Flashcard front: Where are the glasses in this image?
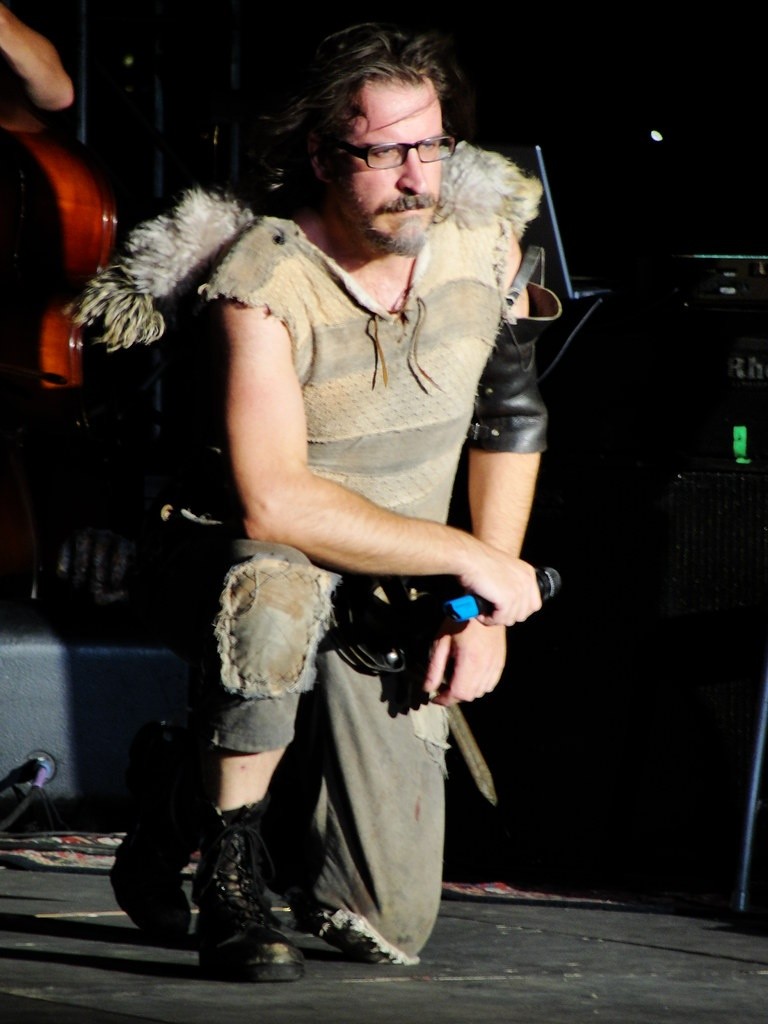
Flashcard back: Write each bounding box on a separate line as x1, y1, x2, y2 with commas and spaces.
331, 129, 456, 170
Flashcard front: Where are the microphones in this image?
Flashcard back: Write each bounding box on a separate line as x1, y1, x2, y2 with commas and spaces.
448, 568, 562, 623
27, 760, 51, 789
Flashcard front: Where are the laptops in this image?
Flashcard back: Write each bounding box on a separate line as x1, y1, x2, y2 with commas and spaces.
505, 145, 610, 300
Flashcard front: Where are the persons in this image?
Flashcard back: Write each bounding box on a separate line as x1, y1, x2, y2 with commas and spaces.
72, 20, 562, 981
0, 1, 75, 134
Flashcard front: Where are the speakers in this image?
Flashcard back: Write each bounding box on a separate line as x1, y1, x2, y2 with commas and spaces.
0, 631, 189, 812
628, 450, 768, 897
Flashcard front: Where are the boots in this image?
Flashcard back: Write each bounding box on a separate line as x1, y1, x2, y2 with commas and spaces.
192, 799, 305, 982
110, 774, 214, 943
